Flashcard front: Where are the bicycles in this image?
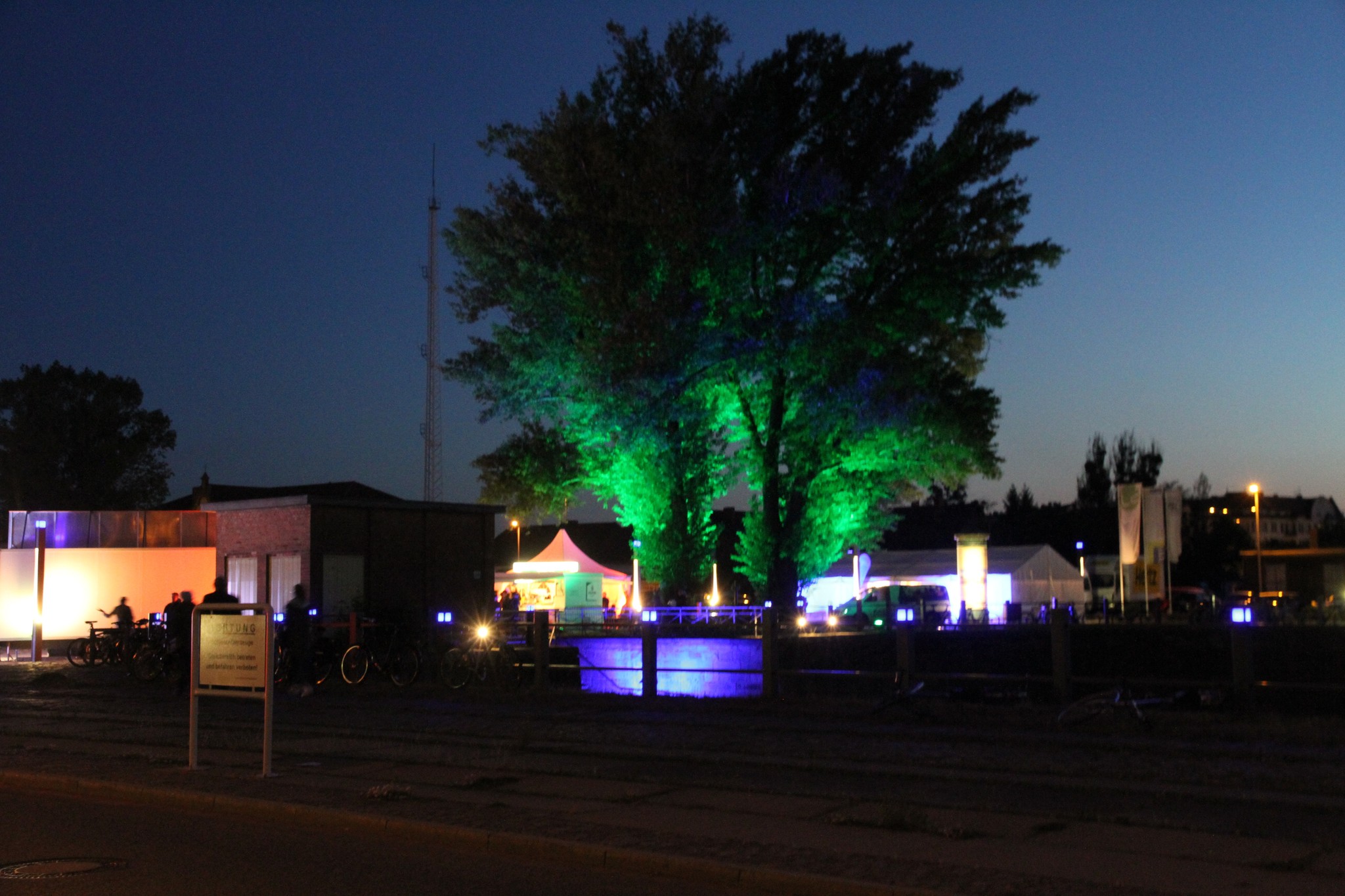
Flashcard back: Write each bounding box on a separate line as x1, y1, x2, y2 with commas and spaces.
66, 619, 525, 691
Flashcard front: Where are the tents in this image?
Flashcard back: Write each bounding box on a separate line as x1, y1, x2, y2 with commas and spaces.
504, 528, 629, 624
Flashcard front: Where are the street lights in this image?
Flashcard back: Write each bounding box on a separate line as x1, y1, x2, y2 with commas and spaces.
1247, 483, 1262, 593
510, 520, 520, 561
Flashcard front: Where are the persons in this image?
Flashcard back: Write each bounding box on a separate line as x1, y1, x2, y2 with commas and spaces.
486, 590, 633, 640
100, 577, 309, 672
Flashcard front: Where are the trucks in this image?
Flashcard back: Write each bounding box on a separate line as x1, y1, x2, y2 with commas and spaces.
1084, 554, 1166, 613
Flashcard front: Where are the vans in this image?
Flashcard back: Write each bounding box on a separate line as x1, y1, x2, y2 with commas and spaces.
832, 585, 952, 629
